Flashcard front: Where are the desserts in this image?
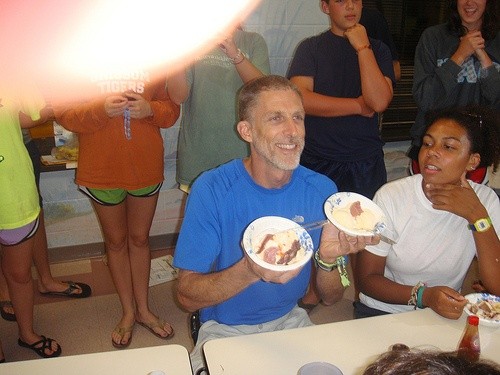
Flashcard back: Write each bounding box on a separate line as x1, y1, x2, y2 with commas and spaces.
332, 201, 379, 232
254, 229, 305, 266
469, 299, 500, 322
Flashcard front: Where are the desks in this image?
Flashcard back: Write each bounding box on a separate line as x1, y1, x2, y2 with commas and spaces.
202, 307, 500, 375
0, 344, 194, 375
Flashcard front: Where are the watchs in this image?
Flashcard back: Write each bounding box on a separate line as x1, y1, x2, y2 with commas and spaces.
467, 216, 492, 232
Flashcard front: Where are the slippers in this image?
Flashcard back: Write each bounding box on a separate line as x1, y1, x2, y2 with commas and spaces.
112, 322, 135, 349
0, 345, 5, 363
40, 281, 92, 297
135, 312, 174, 339
18, 335, 61, 359
0, 300, 17, 322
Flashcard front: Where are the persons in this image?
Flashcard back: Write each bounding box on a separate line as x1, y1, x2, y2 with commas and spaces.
408, 0, 500, 187
0, 90, 84, 362
176, 75, 380, 375
167, 24, 272, 195
289, 0, 394, 200
353, 108, 500, 321
55, 69, 180, 347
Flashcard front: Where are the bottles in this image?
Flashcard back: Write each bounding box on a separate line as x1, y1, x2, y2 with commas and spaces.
456, 315, 481, 362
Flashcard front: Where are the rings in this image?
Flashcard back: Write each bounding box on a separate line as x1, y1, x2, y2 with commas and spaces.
455, 306, 458, 311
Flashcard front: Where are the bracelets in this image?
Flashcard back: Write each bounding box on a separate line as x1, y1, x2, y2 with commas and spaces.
355, 44, 373, 55
230, 48, 245, 64
407, 280, 428, 310
315, 248, 351, 287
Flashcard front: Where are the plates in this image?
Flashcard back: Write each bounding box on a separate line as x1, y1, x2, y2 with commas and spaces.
461, 293, 500, 325
298, 361, 343, 375
244, 215, 313, 271
323, 191, 387, 238
